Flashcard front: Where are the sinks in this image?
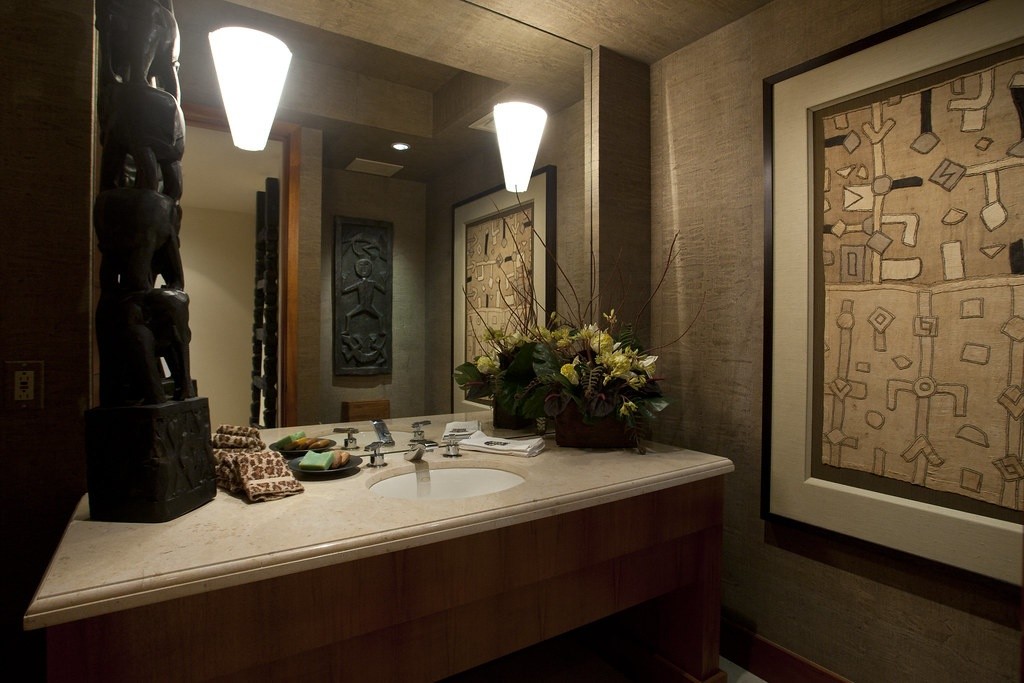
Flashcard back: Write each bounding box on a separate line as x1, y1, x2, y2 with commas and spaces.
317, 429, 418, 458
363, 453, 533, 501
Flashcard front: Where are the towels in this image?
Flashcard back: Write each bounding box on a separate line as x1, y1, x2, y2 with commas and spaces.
218, 452, 306, 502
441, 420, 482, 443
459, 430, 546, 458
212, 423, 266, 486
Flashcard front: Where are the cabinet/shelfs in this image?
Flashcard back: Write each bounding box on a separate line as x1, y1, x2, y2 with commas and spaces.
43, 474, 728, 683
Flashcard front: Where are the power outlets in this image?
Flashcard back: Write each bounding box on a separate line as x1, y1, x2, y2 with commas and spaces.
3, 360, 46, 417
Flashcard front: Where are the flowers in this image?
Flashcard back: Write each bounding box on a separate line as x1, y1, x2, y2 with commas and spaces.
532, 308, 669, 455
452, 320, 565, 417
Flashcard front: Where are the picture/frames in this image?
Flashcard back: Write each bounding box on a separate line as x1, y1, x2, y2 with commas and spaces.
450, 165, 558, 413
760, 0, 1023, 586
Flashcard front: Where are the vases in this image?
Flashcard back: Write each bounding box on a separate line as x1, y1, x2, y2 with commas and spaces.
555, 405, 638, 447
493, 395, 537, 429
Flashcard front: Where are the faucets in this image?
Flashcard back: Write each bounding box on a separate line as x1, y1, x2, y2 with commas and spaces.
404, 421, 439, 461
369, 418, 396, 446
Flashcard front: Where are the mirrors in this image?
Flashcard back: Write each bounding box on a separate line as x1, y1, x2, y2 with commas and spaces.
99, 0, 591, 433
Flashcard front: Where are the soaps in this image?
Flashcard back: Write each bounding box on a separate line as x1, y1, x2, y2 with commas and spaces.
275, 429, 330, 449
298, 450, 350, 470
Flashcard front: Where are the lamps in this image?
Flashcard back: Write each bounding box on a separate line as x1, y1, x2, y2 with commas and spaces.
490, 102, 548, 194
209, 22, 294, 153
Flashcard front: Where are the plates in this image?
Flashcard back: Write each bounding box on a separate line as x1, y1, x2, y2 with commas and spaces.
287, 454, 363, 475
